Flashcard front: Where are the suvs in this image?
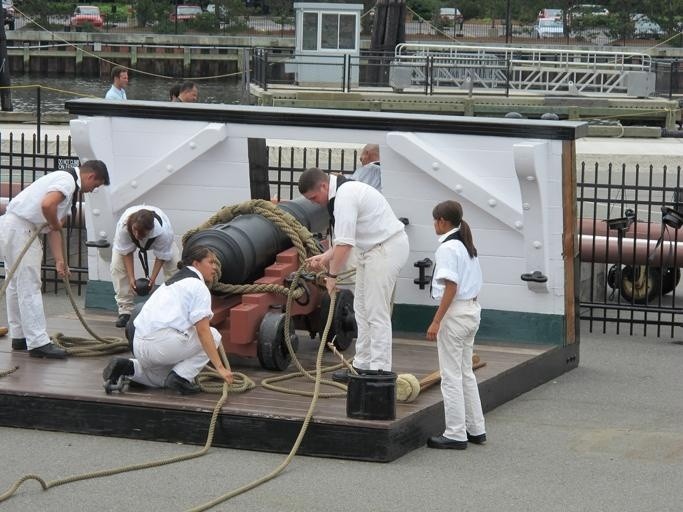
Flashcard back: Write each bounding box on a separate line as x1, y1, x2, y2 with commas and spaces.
65, 5, 103, 32
2, 0, 15, 31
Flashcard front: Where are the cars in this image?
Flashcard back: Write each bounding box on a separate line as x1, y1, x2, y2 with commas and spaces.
168, 3, 230, 30
531, 5, 683, 46
430, 7, 462, 30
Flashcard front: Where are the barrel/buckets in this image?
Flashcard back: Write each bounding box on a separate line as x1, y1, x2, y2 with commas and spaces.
346, 366, 399, 420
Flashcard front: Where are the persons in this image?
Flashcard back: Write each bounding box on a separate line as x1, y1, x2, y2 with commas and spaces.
0, 160, 110, 358
427, 201, 486, 448
103, 248, 233, 394
109, 204, 178, 326
175, 81, 198, 102
170, 83, 182, 101
299, 168, 410, 380
351, 143, 381, 193
105, 65, 128, 100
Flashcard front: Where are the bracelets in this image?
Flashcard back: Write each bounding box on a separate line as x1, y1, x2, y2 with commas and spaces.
326, 272, 338, 279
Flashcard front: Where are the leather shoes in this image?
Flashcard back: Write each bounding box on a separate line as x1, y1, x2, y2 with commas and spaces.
29, 343, 65, 359
332, 367, 361, 383
12, 338, 27, 350
116, 314, 130, 327
103, 358, 123, 381
467, 432, 486, 444
427, 436, 467, 450
164, 374, 201, 394
